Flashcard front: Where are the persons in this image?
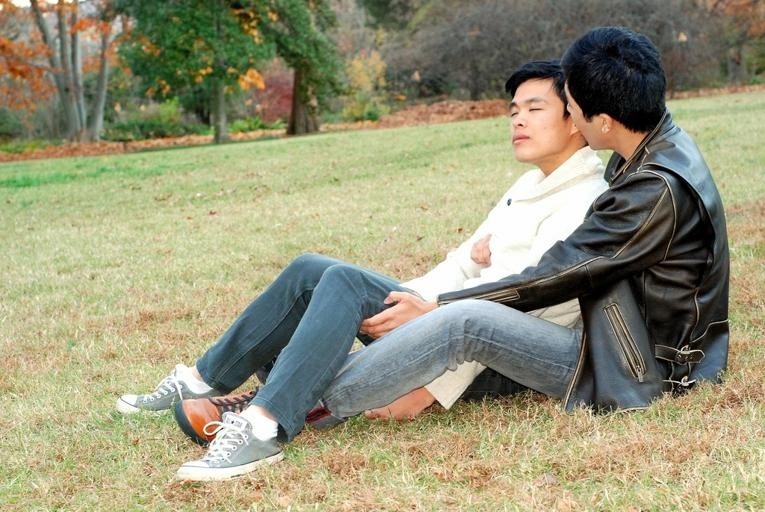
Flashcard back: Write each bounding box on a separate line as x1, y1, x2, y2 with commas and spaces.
114, 57, 614, 482
172, 23, 733, 448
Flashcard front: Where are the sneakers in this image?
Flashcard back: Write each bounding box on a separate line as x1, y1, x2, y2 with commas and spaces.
175, 387, 263, 449
117, 364, 223, 416
177, 412, 285, 481
